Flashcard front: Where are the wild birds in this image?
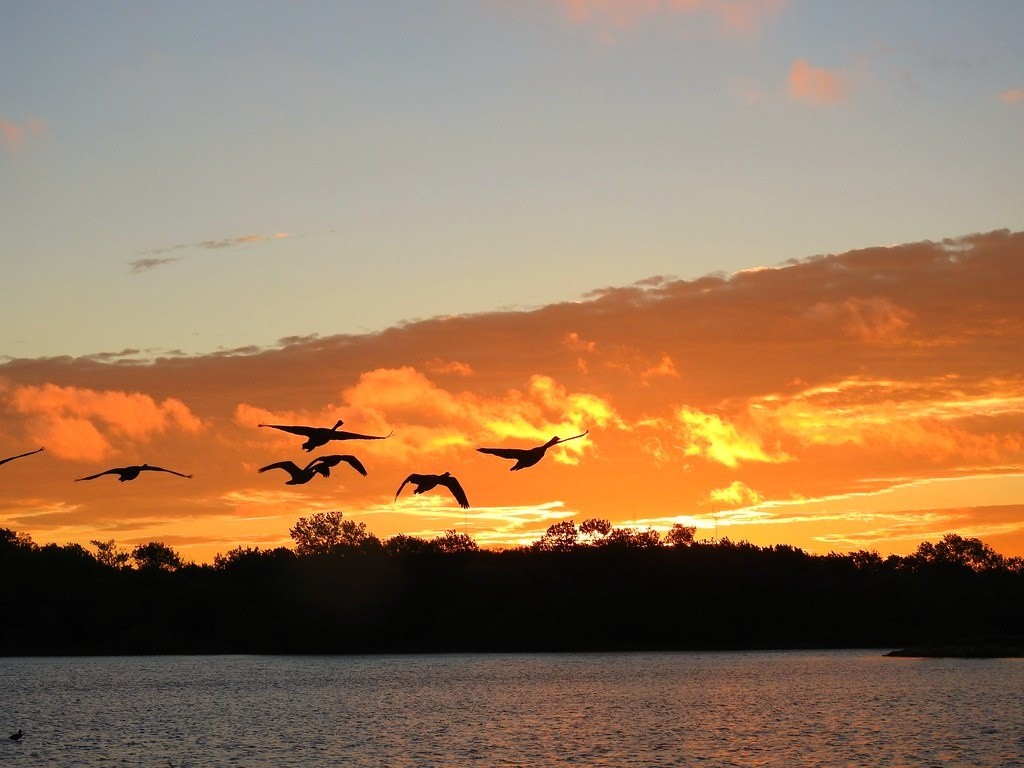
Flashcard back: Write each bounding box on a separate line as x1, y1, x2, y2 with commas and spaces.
0, 446, 45, 464
8, 729, 24, 740
74, 464, 192, 482
394, 471, 470, 510
258, 419, 396, 452
303, 455, 368, 477
474, 429, 591, 472
258, 460, 331, 487
